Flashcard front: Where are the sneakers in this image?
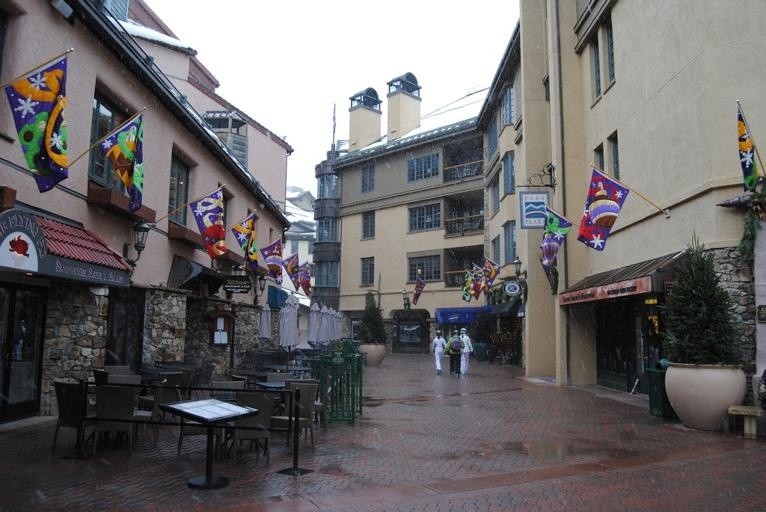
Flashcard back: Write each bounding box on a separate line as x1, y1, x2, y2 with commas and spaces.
450, 372, 454, 375
436, 370, 439, 375
456, 374, 460, 378
439, 370, 442, 373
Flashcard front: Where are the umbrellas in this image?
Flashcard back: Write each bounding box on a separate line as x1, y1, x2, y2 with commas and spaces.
257, 290, 344, 369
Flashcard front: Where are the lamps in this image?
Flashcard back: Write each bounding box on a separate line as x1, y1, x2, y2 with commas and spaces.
254, 270, 270, 296
123, 219, 150, 265
513, 256, 522, 275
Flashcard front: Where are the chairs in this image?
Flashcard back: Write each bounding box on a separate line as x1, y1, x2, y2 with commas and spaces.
52, 364, 319, 489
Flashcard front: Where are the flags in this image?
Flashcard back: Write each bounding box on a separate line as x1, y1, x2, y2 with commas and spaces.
5, 53, 68, 192
737, 105, 762, 194
231, 213, 262, 270
259, 238, 283, 285
188, 187, 228, 259
466, 264, 483, 300
574, 167, 629, 251
96, 110, 143, 213
460, 269, 473, 302
480, 258, 502, 293
538, 207, 572, 297
411, 274, 425, 306
299, 262, 311, 296
281, 252, 300, 291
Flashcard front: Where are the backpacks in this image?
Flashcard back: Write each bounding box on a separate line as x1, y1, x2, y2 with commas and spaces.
450, 337, 461, 352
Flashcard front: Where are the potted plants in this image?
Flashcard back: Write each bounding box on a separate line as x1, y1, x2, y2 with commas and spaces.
658, 229, 748, 434
357, 289, 387, 367
642, 316, 668, 416
469, 308, 493, 361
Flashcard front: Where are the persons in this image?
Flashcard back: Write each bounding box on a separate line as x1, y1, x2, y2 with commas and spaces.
444, 329, 466, 380
431, 330, 448, 376
459, 327, 474, 376
